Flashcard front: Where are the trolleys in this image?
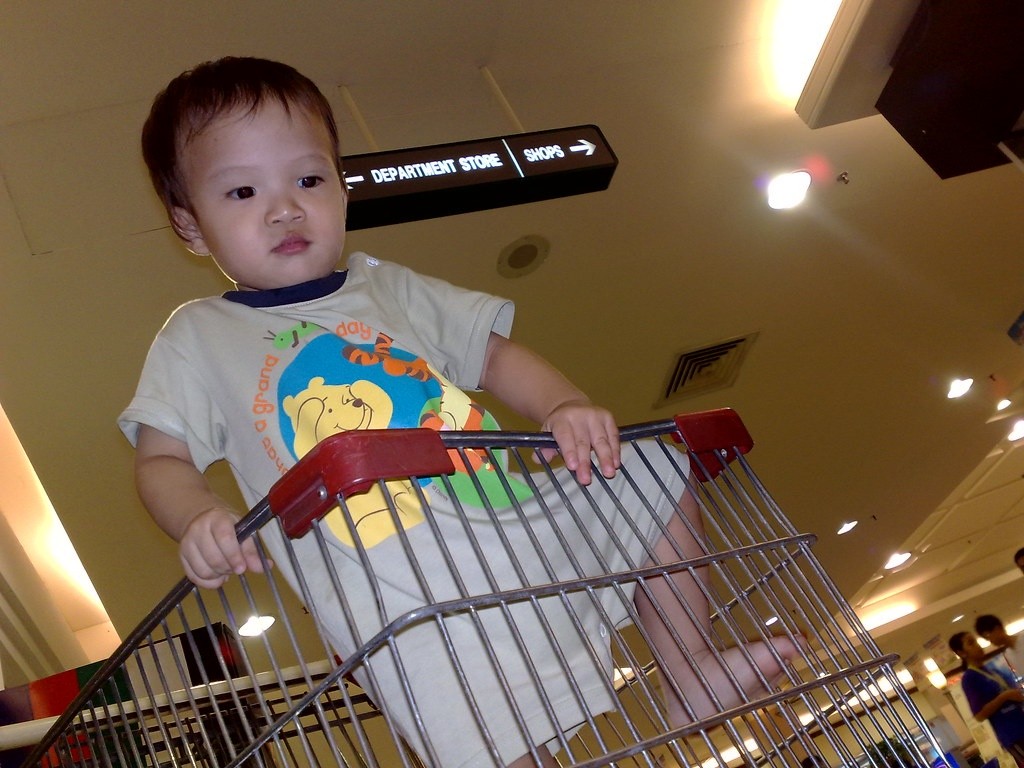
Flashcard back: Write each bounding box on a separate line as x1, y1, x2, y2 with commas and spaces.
18, 406, 953, 768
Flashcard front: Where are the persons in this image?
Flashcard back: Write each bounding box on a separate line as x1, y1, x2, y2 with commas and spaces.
802, 755, 827, 768
115, 56, 805, 768
1013, 546, 1024, 571
972, 614, 1024, 677
947, 631, 1024, 768
